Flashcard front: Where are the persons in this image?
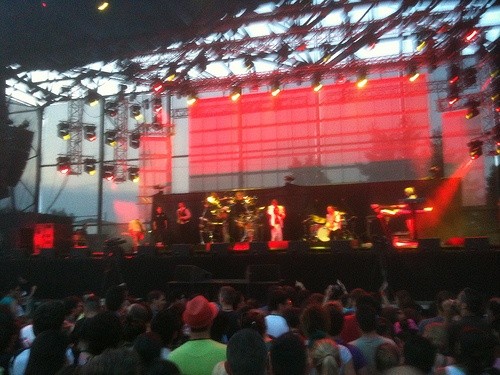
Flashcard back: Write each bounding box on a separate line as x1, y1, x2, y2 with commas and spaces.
0, 284, 500, 375
404, 187, 419, 198
324, 204, 337, 223
126, 198, 235, 247
265, 199, 286, 242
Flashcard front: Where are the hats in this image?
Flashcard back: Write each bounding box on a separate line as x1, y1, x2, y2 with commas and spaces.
182, 296, 219, 327
264, 315, 289, 338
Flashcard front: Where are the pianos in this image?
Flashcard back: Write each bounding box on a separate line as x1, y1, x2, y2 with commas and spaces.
373, 204, 434, 239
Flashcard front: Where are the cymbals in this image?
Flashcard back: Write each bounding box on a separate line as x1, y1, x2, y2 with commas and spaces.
332, 211, 347, 215
308, 214, 327, 222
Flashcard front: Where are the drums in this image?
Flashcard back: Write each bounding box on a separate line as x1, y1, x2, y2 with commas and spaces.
317, 228, 331, 241
308, 224, 322, 237
327, 217, 341, 231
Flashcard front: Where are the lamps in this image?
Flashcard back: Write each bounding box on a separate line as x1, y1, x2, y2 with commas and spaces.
58, 0, 500, 185
